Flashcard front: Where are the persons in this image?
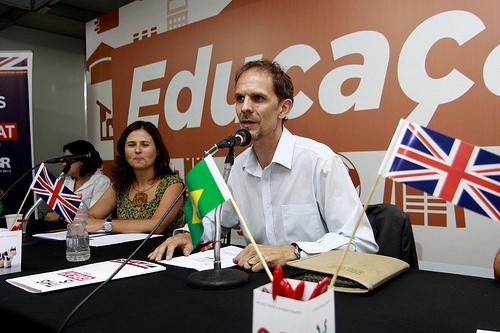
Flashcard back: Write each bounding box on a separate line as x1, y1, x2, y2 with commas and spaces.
86, 121, 186, 235
148, 60, 379, 272
45, 140, 112, 221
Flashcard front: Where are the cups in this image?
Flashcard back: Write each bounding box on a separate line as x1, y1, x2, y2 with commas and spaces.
5, 214, 23, 233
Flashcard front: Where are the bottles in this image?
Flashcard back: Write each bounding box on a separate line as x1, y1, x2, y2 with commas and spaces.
65, 193, 91, 262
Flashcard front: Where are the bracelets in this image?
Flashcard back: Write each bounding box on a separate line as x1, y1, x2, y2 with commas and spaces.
58, 216, 63, 220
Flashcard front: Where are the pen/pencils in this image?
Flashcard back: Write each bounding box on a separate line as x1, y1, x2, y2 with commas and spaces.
310, 276, 330, 301
270, 263, 306, 301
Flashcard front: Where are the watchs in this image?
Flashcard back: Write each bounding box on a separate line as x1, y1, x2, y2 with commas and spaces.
103, 219, 113, 233
291, 243, 302, 259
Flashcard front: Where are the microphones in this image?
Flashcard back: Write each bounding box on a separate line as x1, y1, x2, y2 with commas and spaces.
202, 128, 252, 160
44, 151, 95, 163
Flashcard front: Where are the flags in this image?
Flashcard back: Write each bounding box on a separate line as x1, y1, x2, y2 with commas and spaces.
32, 166, 82, 223
379, 119, 500, 222
184, 156, 232, 247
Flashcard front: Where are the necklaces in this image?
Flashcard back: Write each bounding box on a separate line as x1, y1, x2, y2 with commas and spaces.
132, 179, 157, 207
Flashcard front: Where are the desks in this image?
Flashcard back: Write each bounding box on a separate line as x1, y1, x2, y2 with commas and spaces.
0, 225, 500, 333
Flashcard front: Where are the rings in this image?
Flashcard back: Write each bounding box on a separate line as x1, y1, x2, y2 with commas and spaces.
248, 262, 255, 266
